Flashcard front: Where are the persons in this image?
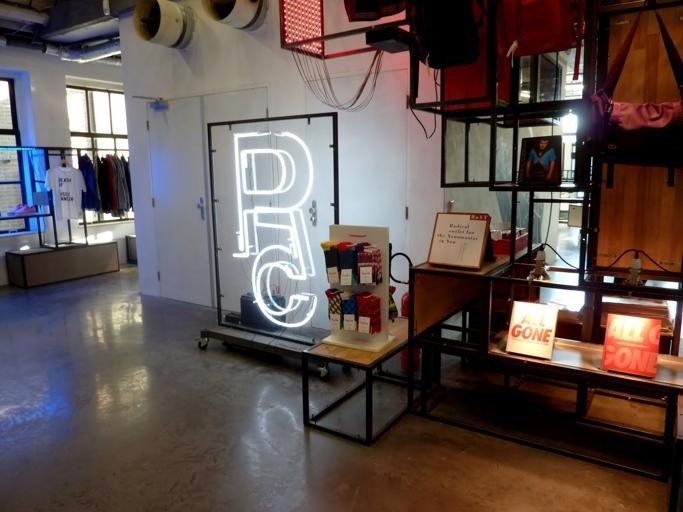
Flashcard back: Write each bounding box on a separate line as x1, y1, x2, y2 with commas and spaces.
523, 136, 557, 183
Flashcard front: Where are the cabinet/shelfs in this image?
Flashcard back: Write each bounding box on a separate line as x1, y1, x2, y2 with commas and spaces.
300, 317, 420, 447
405, 1, 682, 484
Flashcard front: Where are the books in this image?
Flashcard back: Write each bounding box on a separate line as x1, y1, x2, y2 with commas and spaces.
504, 299, 560, 361
598, 313, 662, 379
599, 296, 671, 333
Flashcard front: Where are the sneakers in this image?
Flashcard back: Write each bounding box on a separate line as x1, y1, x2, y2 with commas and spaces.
7, 204, 23, 214
15, 205, 36, 214
